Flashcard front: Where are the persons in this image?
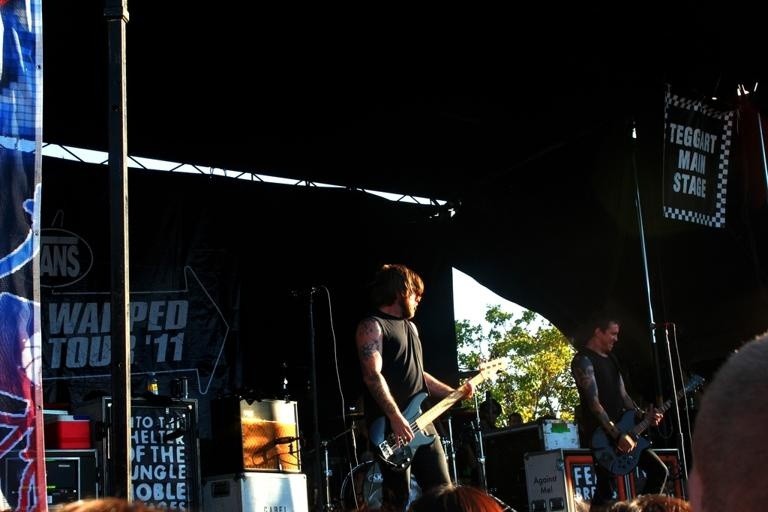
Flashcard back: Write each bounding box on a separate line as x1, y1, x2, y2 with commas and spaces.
413, 485, 507, 512
57, 498, 166, 512
688, 331, 768, 512
509, 413, 523, 426
609, 493, 690, 512
462, 399, 502, 484
356, 264, 477, 512
571, 313, 668, 512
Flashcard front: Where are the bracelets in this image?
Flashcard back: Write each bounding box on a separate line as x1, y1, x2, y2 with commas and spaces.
616, 432, 622, 444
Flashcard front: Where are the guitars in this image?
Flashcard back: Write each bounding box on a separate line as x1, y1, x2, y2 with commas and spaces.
590, 374, 706, 475
369, 358, 507, 473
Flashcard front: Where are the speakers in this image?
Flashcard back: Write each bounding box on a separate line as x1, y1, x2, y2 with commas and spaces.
106, 396, 199, 512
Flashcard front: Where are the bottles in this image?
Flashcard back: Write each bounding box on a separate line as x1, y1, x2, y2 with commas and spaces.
147, 372, 159, 395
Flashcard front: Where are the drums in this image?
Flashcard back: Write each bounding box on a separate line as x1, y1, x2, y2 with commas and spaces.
340, 460, 423, 512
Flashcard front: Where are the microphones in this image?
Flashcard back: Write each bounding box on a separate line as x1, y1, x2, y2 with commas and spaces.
649, 323, 674, 330
286, 285, 322, 299
